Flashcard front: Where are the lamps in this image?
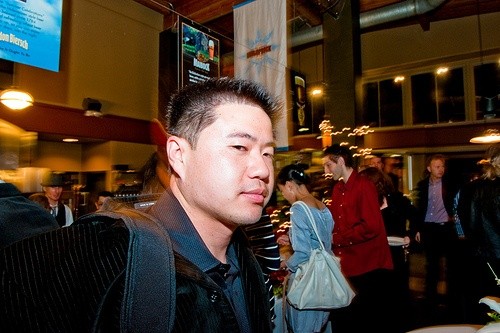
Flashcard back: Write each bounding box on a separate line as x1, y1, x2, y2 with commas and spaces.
469, 125, 500, 144
82, 99, 102, 118
1, 61, 34, 112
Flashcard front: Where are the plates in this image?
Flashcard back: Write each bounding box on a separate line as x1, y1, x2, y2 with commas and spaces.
387, 237, 408, 246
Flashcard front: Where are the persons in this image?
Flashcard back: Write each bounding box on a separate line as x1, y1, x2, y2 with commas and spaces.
369, 157, 385, 170
242, 208, 280, 325
453, 172, 480, 238
42, 173, 74, 227
359, 167, 420, 332
322, 145, 393, 332
357, 165, 369, 174
91, 191, 114, 212
415, 154, 462, 316
0, 79, 283, 333
0, 179, 60, 243
277, 162, 335, 333
388, 163, 404, 189
458, 142, 500, 325
29, 194, 51, 215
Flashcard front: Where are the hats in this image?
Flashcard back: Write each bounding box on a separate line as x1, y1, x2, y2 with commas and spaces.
40, 172, 65, 186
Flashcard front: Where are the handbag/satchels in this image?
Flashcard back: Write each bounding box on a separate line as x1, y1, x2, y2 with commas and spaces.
286, 249, 356, 309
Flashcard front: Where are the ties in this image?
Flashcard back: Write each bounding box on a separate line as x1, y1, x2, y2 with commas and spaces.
52, 207, 56, 217
